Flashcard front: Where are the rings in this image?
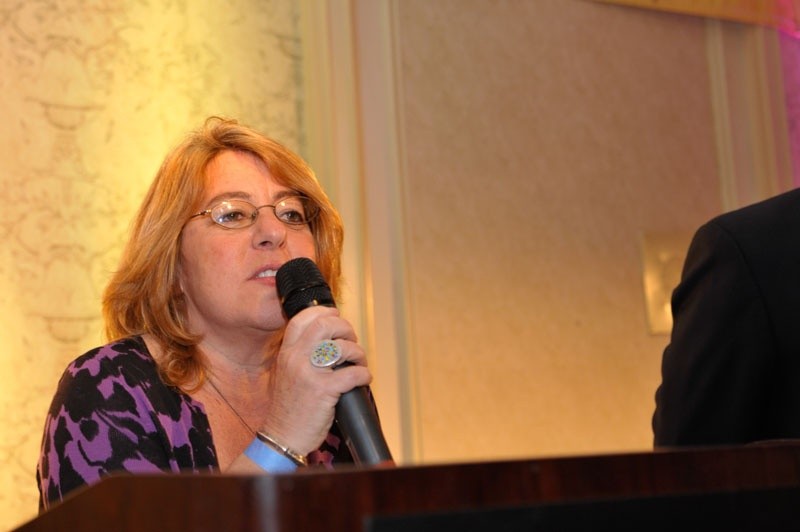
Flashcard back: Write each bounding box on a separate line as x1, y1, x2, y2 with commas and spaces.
311, 339, 341, 368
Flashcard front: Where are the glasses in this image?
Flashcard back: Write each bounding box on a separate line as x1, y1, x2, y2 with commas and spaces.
189, 196, 320, 229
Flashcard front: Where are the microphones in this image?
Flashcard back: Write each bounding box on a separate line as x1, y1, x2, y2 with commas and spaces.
276, 257, 395, 469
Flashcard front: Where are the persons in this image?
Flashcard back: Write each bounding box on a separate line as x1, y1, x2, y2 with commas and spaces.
36, 115, 357, 515
652, 188, 800, 453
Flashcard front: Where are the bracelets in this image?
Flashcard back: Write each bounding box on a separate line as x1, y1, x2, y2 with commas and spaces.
255, 430, 307, 467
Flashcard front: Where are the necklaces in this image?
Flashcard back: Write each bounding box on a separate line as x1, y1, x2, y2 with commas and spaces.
205, 374, 256, 436
